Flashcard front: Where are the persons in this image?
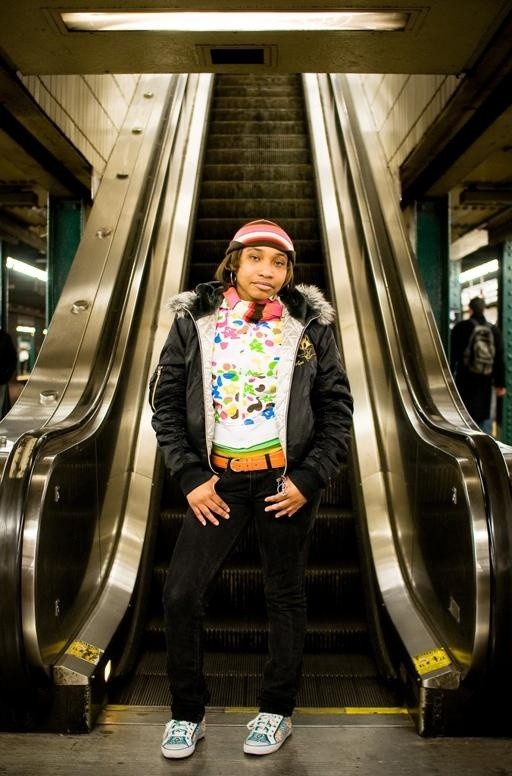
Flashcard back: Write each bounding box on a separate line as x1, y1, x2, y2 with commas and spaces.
448, 296, 507, 435
145, 213, 354, 763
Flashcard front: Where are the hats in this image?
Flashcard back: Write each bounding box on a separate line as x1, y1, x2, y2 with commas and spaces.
224, 218, 296, 267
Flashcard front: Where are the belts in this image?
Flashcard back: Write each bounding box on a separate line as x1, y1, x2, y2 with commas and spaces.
212, 449, 286, 473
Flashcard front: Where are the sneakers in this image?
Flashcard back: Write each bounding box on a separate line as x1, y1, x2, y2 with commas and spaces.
161, 714, 206, 760
243, 712, 293, 756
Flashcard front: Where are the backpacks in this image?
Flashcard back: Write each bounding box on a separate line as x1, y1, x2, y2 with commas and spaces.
463, 319, 496, 375
